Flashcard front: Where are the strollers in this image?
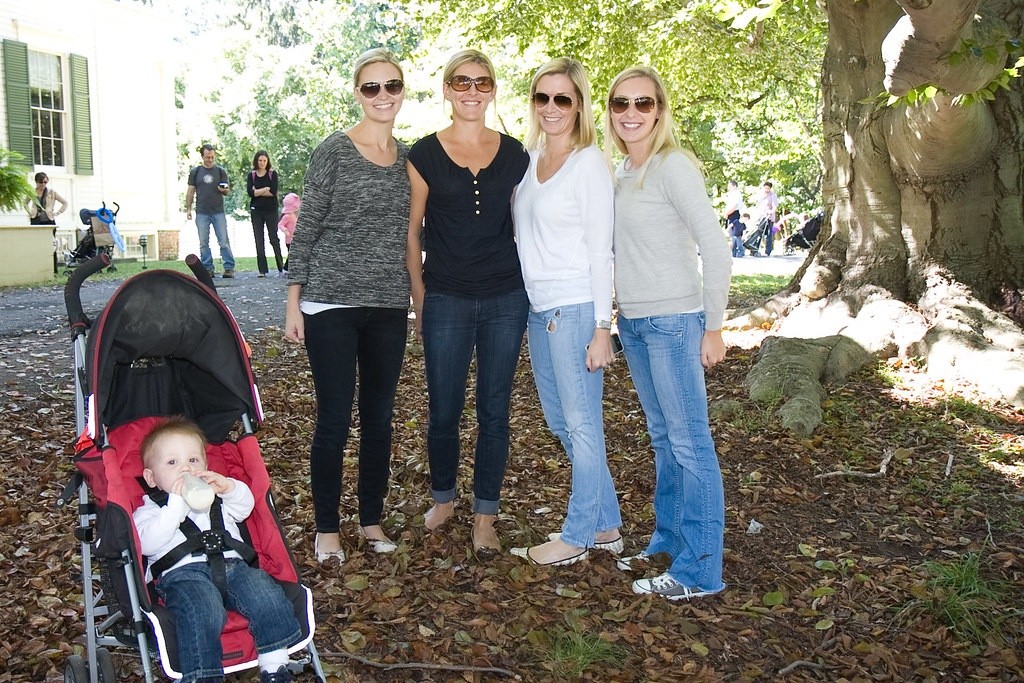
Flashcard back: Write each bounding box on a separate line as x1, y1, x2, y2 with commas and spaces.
781, 210, 824, 257
60, 201, 120, 276
64, 252, 328, 683
734, 212, 773, 258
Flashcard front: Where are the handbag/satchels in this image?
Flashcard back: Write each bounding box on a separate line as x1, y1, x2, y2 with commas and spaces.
90, 212, 116, 247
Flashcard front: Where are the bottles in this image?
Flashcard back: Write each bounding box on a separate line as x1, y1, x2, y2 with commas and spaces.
179, 471, 215, 511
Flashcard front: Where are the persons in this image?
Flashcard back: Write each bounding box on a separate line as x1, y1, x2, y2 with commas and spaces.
510, 56, 624, 567
719, 179, 825, 258
278, 193, 301, 275
24, 173, 68, 276
247, 150, 286, 277
132, 419, 301, 683
284, 48, 410, 567
406, 50, 531, 563
604, 66, 732, 602
186, 145, 235, 278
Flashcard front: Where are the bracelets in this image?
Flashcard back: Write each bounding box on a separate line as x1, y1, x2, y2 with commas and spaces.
263, 188, 267, 192
705, 330, 722, 334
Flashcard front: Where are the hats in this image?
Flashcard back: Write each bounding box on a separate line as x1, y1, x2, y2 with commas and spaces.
282, 193, 300, 213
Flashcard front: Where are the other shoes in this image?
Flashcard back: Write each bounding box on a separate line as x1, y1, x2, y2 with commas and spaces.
261, 663, 295, 683
257, 271, 269, 276
548, 533, 624, 554
417, 506, 454, 533
471, 526, 500, 558
359, 524, 397, 553
510, 541, 590, 566
315, 532, 345, 566
279, 269, 289, 275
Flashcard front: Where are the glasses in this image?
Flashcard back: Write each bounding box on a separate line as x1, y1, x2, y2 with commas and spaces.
610, 96, 660, 113
446, 75, 495, 93
359, 79, 404, 98
531, 93, 581, 112
38, 180, 48, 184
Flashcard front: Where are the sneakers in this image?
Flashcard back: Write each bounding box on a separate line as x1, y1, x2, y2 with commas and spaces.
632, 573, 715, 601
617, 552, 670, 571
223, 269, 235, 277
207, 270, 215, 277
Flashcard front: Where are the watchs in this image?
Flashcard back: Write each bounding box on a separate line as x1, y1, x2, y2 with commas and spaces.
594, 320, 611, 329
